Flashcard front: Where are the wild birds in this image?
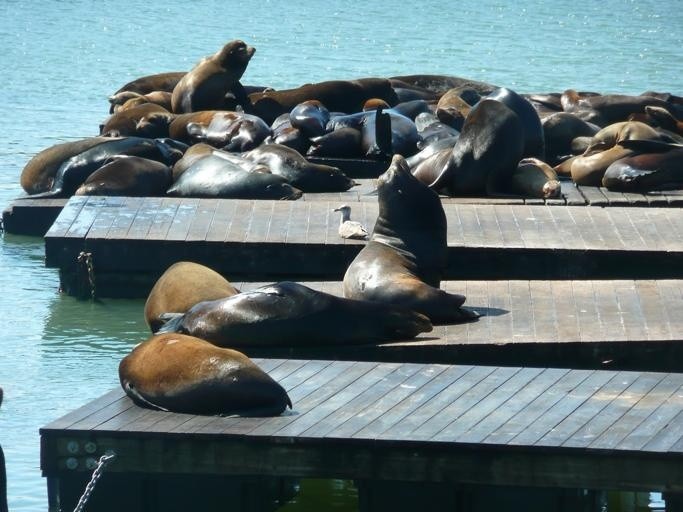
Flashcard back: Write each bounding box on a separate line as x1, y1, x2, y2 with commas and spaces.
334, 205, 369, 239
